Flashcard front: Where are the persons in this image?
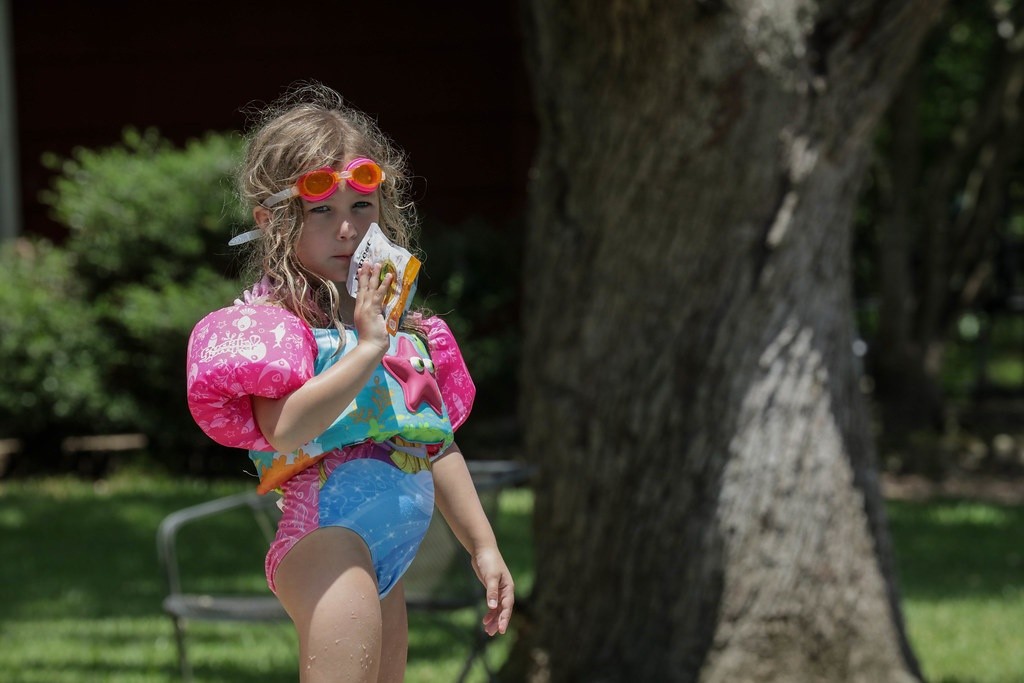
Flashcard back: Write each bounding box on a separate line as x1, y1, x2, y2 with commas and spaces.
186, 103, 516, 683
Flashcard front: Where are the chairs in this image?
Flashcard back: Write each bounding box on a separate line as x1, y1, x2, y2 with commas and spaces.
158, 453, 517, 683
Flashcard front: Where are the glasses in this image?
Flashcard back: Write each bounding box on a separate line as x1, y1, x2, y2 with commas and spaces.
262, 155, 386, 207
